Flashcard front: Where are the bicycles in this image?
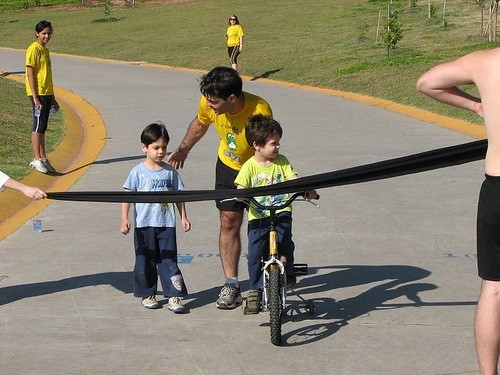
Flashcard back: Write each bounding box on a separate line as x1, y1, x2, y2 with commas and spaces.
220, 192, 320, 345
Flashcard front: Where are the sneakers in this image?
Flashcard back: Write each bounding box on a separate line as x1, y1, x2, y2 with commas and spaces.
141, 296, 161, 310
29, 158, 48, 174
168, 296, 186, 314
217, 286, 243, 310
42, 159, 56, 173
244, 289, 261, 315
281, 275, 296, 288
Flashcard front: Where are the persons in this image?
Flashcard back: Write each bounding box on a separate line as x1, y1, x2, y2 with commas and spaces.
416, 48, 500, 375
167, 67, 274, 309
0, 171, 47, 201
224, 15, 244, 70
26, 21, 60, 173
234, 113, 317, 314
120, 123, 191, 313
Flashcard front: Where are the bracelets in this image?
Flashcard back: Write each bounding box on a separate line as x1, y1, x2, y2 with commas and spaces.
240, 45, 243, 46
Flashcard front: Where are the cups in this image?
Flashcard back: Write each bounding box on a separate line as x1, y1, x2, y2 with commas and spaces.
35, 108, 40, 116
33, 220, 42, 233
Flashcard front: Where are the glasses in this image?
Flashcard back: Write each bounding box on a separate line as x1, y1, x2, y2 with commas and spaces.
230, 18, 237, 21
